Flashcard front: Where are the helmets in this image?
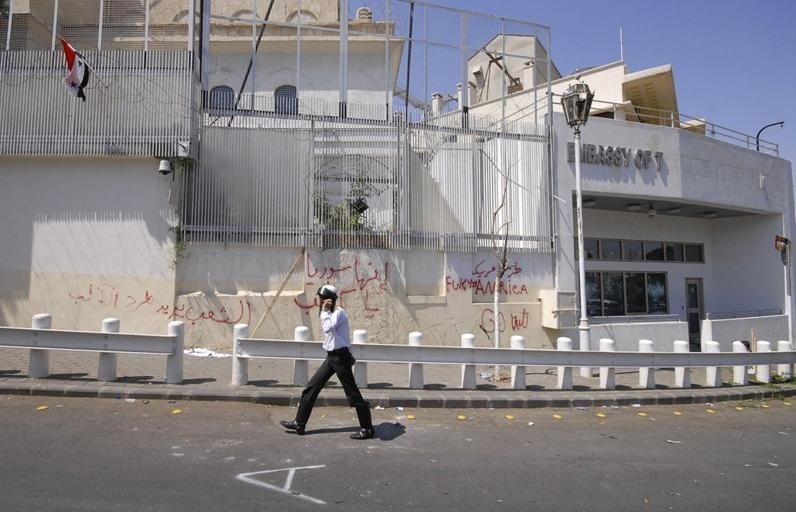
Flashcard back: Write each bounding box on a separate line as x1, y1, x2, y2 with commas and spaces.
317, 285, 338, 300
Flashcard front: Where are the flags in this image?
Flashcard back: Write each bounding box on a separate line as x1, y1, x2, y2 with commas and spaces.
61, 40, 88, 102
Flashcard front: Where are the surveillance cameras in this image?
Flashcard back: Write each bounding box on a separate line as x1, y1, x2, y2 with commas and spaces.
158, 160, 172, 176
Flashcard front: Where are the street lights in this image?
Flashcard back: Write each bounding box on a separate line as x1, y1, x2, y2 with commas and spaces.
559, 78, 597, 377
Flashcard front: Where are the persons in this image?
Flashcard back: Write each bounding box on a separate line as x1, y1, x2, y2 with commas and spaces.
281, 285, 374, 440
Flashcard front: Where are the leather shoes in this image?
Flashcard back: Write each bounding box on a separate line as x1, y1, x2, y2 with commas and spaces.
280, 420, 306, 435
350, 428, 375, 440
